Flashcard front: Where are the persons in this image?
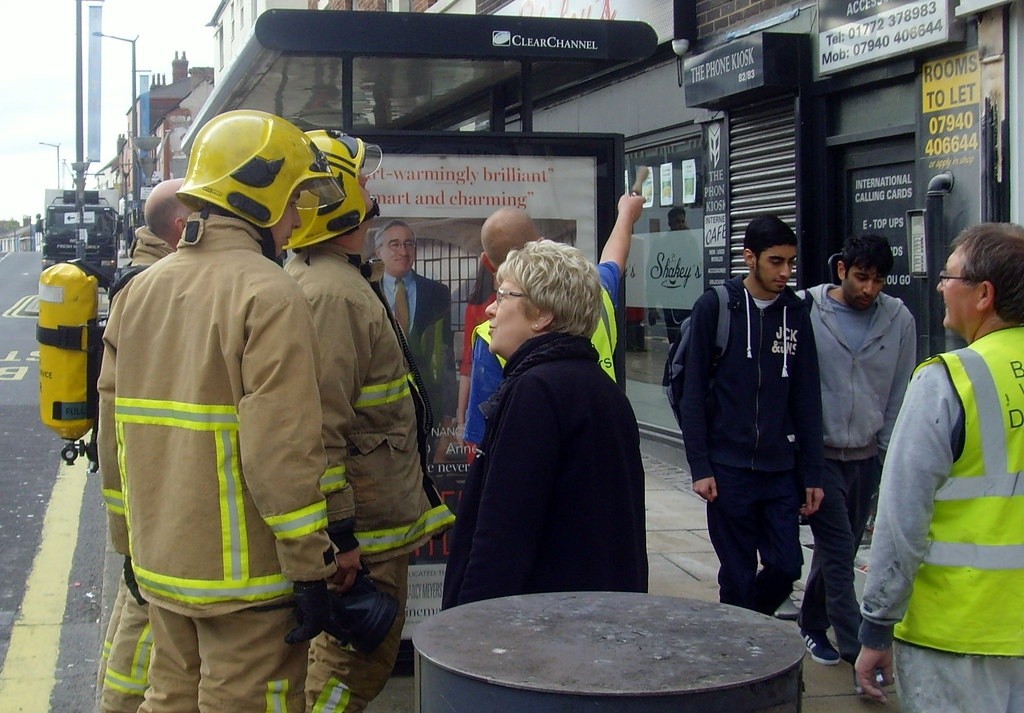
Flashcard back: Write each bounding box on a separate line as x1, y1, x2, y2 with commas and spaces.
440, 234, 652, 608
94, 107, 341, 713
280, 129, 458, 713
792, 234, 917, 662
454, 251, 501, 426
854, 222, 1024, 713
367, 221, 459, 467
461, 192, 646, 444
678, 214, 827, 614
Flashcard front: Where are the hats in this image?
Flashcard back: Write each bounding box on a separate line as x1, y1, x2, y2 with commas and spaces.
743, 212, 797, 257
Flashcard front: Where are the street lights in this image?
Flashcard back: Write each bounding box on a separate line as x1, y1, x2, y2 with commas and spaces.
39, 142, 60, 189
92, 31, 143, 228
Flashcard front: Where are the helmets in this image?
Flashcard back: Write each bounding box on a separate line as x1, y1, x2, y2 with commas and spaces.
175, 108, 337, 227
280, 129, 366, 249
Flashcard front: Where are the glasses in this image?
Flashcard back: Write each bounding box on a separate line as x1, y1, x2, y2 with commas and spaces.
939, 267, 985, 290
496, 286, 527, 306
380, 239, 416, 253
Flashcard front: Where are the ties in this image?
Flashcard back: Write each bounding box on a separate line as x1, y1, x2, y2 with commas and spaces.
395, 277, 410, 340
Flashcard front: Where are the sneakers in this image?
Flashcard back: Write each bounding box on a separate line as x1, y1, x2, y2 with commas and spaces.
800, 630, 842, 665
838, 651, 884, 683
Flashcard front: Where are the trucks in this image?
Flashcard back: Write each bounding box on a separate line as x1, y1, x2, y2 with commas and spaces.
35, 189, 123, 288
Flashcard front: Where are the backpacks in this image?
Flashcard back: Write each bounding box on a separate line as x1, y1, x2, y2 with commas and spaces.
661, 281, 731, 445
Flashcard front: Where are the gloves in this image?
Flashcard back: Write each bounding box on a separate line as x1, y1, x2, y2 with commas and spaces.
284, 569, 328, 647
122, 554, 145, 606
320, 562, 401, 661
